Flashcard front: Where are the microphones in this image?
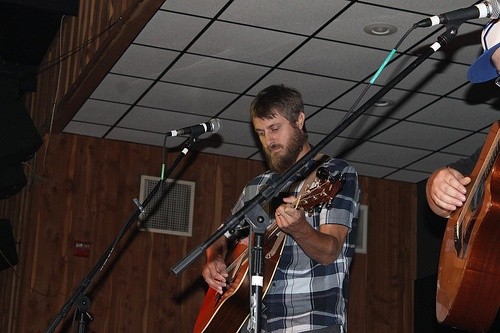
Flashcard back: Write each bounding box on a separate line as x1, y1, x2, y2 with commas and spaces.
417, 0, 500, 27
168, 118, 223, 136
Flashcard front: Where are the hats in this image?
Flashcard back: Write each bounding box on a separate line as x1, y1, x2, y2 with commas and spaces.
467, 17, 500, 83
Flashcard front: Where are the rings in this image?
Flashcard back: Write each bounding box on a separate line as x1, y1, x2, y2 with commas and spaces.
277, 213, 281, 217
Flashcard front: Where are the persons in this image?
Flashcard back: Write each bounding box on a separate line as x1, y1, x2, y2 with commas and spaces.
200, 84, 361, 332
424, 19, 500, 222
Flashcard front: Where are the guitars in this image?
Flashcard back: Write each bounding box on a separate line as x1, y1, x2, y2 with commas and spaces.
435, 120, 500, 325
193, 174, 344, 333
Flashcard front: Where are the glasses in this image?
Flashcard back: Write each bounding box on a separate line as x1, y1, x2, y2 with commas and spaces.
495, 77, 500, 87
